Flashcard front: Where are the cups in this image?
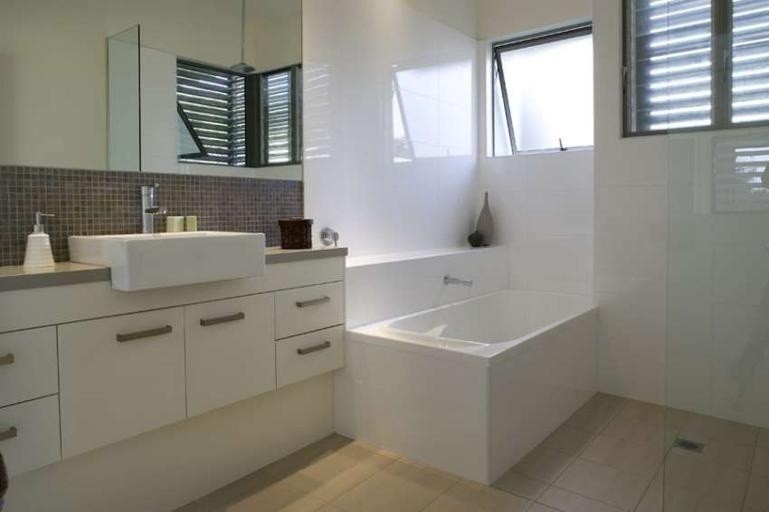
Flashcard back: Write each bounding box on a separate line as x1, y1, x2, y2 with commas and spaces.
185, 215, 198, 233
165, 214, 185, 233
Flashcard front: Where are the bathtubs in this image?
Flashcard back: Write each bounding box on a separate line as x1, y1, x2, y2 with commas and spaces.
334, 283, 601, 487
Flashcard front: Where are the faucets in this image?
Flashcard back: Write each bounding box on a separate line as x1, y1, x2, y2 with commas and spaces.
444, 274, 476, 292
140, 183, 170, 234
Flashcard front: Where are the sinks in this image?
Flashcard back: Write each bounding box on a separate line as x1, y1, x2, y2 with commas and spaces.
67, 229, 265, 293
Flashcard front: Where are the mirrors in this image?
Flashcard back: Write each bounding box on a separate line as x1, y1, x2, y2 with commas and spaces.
1, 1, 303, 185
